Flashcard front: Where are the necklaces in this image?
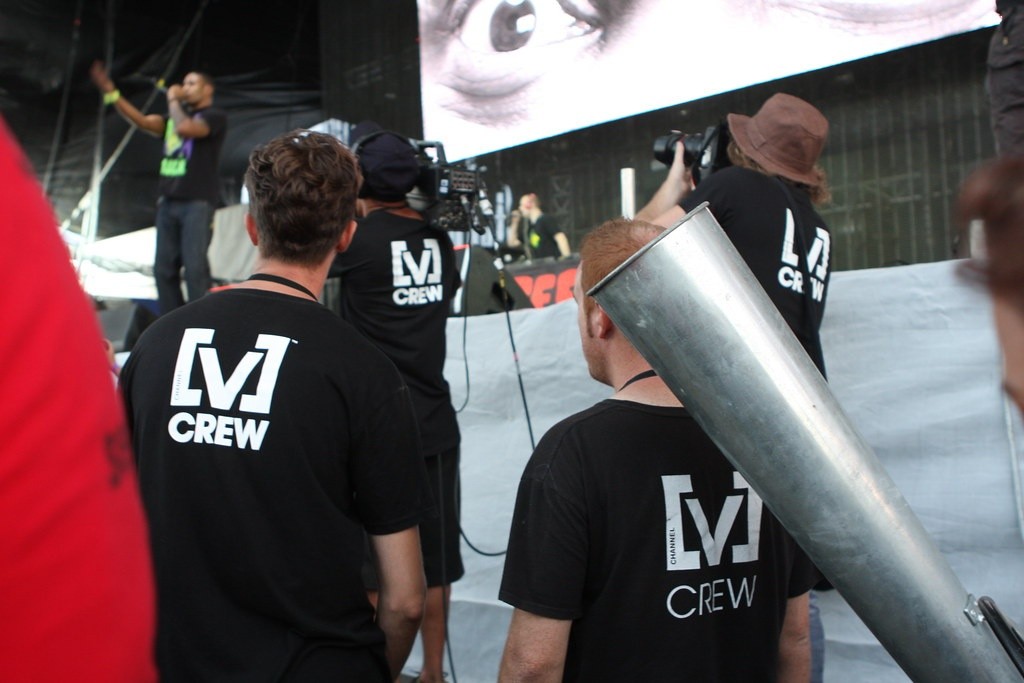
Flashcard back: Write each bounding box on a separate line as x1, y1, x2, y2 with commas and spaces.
616, 367, 660, 394
245, 271, 321, 306
382, 201, 412, 212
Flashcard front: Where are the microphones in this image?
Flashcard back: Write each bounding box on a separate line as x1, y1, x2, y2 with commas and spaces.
155, 77, 172, 89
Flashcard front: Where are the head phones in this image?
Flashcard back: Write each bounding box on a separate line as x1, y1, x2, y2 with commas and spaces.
351, 130, 425, 175
525, 193, 535, 209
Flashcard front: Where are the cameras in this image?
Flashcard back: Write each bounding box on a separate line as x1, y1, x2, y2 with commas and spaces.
654, 121, 732, 182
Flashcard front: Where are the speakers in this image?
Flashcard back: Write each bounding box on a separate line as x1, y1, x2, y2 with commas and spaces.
448, 246, 536, 318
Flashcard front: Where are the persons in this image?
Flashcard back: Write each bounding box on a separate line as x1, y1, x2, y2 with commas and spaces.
416, 0, 991, 161
504, 193, 571, 263
320, 131, 466, 682
957, 147, 1024, 428
120, 126, 429, 683
491, 216, 826, 683
89, 61, 224, 317
629, 88, 834, 683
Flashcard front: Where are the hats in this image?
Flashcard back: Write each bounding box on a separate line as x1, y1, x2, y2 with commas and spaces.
727, 92, 829, 187
347, 120, 422, 203
